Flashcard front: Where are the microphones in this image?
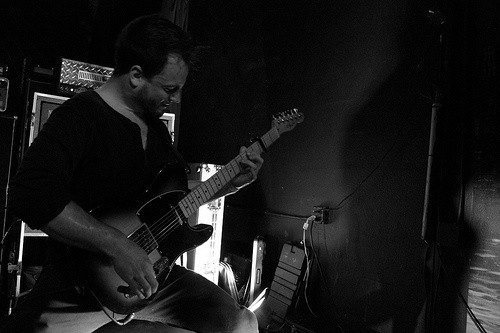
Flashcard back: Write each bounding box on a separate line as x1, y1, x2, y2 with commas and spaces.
424, 5, 449, 26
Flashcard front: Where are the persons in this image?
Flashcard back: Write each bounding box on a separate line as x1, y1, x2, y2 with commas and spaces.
5, 38, 263, 333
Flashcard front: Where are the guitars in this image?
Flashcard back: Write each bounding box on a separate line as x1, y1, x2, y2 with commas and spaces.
81, 104, 305, 314
